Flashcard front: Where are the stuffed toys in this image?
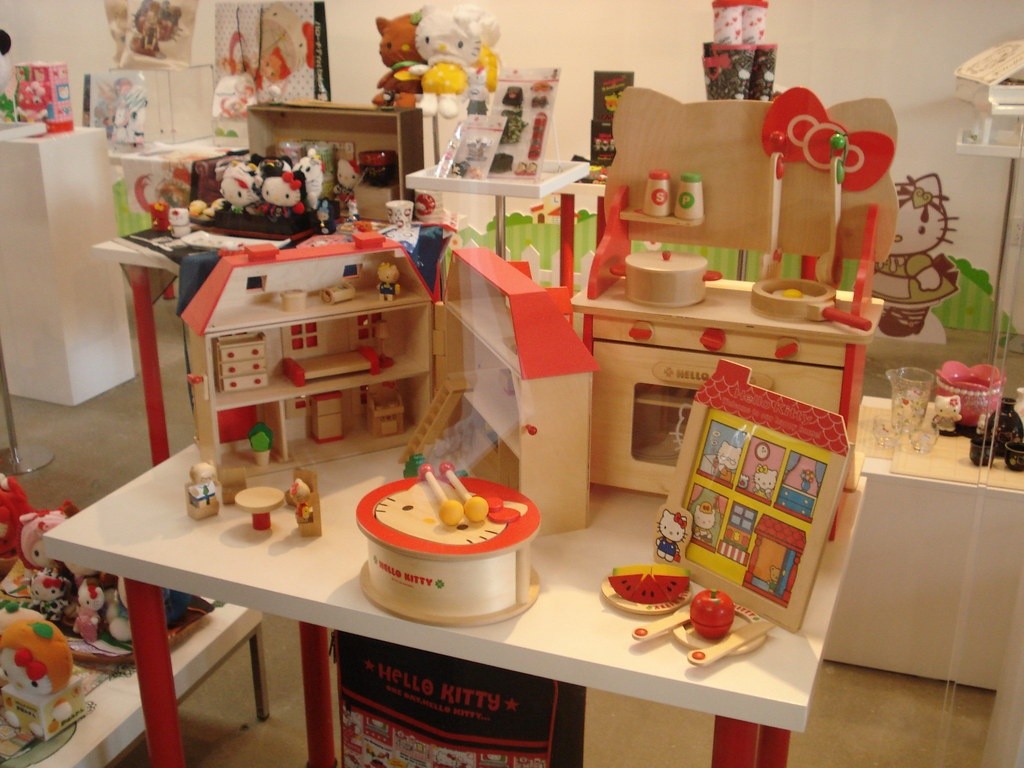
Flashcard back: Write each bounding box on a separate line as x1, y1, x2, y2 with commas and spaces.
186, 147, 363, 235
0, 473, 191, 737
372, 3, 502, 118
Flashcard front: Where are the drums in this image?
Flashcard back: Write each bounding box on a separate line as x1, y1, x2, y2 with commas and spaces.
354, 475, 544, 630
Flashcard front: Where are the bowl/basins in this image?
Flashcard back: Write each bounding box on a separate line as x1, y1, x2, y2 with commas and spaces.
359, 149, 395, 186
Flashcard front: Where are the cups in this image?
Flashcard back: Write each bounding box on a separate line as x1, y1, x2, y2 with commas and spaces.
1004, 442, 1024, 471
674, 173, 704, 218
885, 367, 935, 432
871, 419, 938, 451
969, 438, 999, 466
642, 170, 671, 216
703, 0, 777, 101
385, 200, 414, 230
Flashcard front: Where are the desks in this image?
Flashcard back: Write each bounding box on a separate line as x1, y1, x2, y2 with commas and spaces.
821, 395, 1024, 691
0, 126, 135, 409
42, 442, 867, 768
0, 595, 269, 768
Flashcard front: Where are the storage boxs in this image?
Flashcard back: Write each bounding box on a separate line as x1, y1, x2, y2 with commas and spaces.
17, 61, 73, 134
243, 102, 424, 224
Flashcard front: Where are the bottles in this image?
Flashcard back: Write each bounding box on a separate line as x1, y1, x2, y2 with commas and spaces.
987, 398, 1024, 457
